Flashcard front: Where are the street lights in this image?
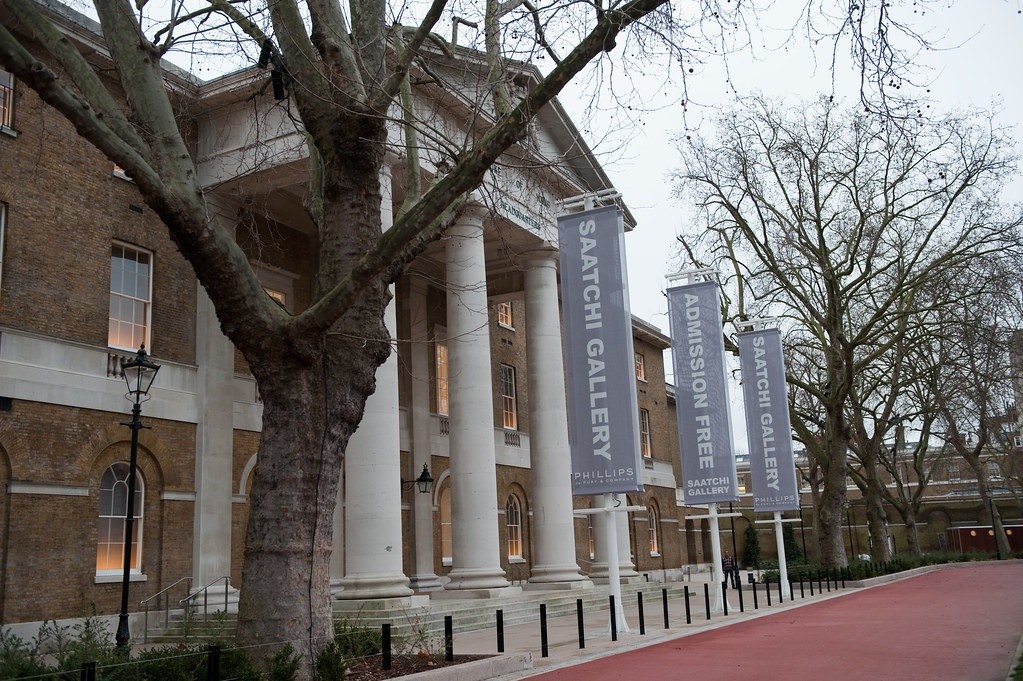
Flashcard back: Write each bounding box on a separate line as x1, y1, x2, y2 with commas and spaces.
112, 342, 162, 660
797, 487, 807, 562
843, 496, 854, 560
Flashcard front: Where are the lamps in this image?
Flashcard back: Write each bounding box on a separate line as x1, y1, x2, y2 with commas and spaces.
400, 462, 435, 499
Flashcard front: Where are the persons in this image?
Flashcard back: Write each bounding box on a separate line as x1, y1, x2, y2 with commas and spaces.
722, 550, 736, 589
